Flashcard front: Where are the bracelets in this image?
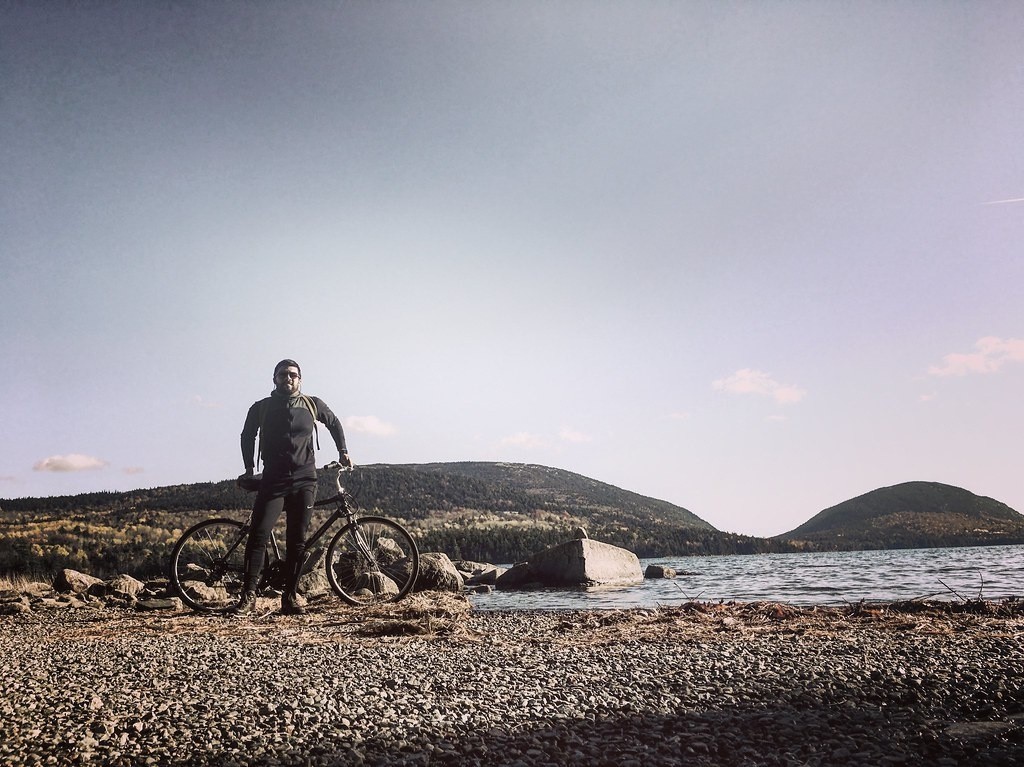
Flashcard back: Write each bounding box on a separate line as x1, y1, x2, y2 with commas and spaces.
339, 450, 348, 454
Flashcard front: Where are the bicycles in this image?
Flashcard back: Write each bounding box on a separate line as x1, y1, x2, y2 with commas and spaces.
168, 461, 418, 618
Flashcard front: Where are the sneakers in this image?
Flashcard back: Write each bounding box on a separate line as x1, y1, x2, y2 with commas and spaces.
233, 591, 256, 614
281, 592, 305, 613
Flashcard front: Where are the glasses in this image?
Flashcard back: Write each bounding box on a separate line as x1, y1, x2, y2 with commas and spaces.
275, 372, 300, 379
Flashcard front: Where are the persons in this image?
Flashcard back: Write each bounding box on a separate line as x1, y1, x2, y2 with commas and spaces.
236, 359, 354, 615
